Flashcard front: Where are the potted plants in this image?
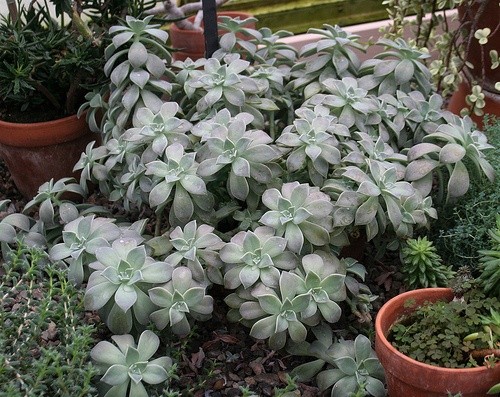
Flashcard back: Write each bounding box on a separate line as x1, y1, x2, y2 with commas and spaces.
0, 0, 113, 203
146, 0, 259, 71
374, 266, 500, 397
362, 0, 500, 135
